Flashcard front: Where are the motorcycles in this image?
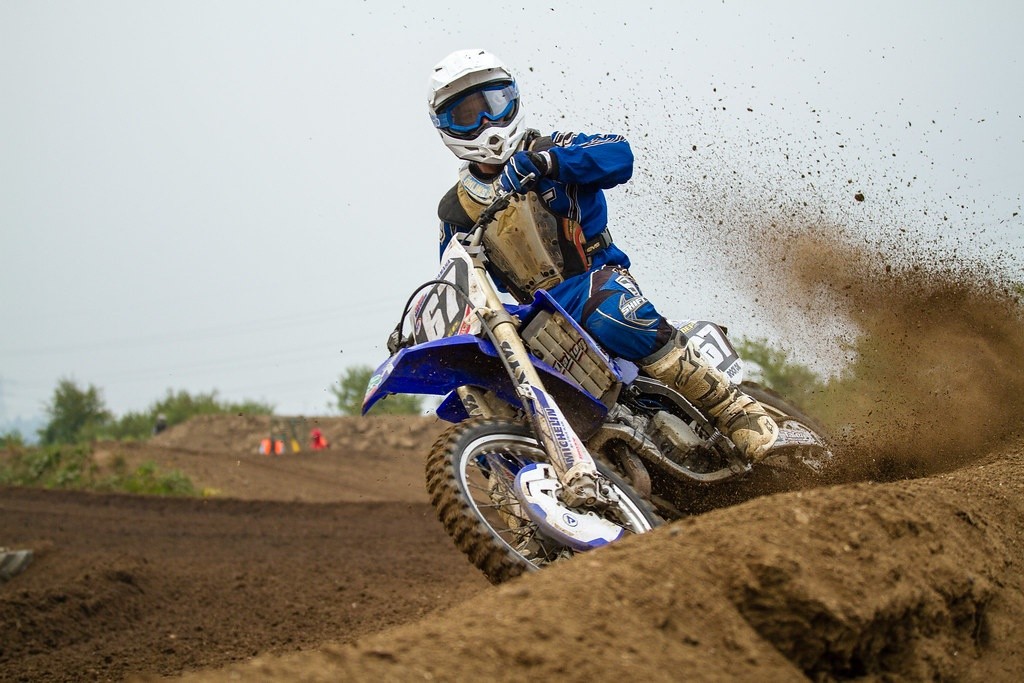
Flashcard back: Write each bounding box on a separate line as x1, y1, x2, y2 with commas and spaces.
360, 168, 844, 587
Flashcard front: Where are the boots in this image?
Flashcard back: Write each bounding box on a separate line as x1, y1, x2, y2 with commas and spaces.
487, 469, 547, 556
640, 325, 780, 464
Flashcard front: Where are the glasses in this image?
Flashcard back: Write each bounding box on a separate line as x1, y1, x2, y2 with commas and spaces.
431, 78, 520, 141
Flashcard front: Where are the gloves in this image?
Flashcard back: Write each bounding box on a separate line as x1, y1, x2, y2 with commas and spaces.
497, 150, 553, 199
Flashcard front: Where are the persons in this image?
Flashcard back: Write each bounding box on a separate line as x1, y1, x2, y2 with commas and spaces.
152, 412, 168, 437
428, 50, 779, 466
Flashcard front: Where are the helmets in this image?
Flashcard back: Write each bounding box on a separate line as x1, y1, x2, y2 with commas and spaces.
426, 47, 527, 164
